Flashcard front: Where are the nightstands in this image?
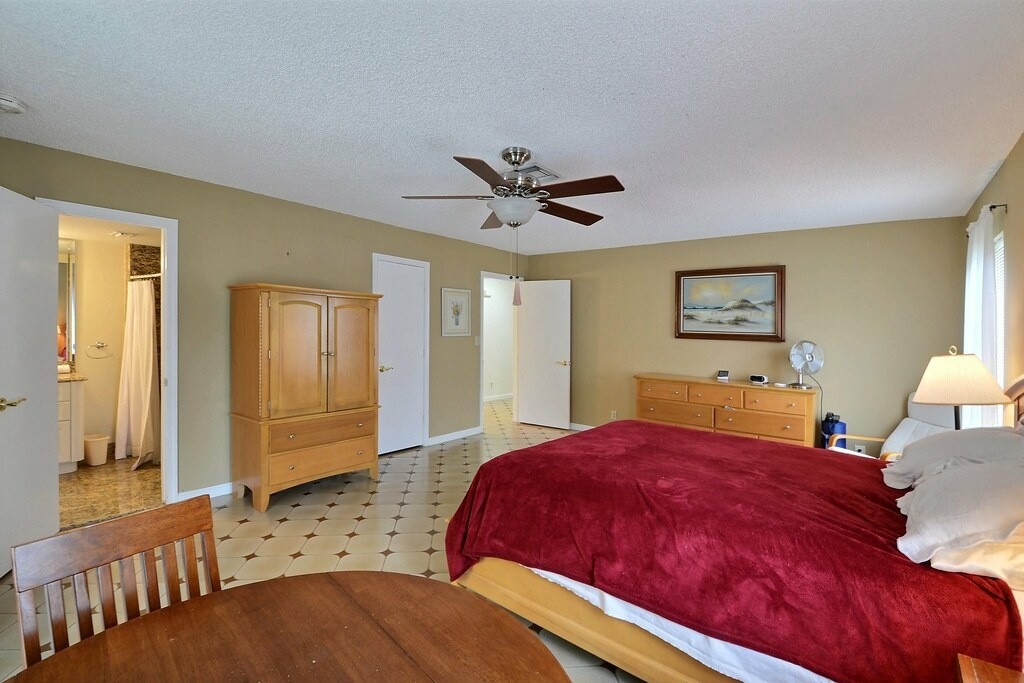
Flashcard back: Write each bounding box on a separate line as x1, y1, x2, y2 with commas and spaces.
633, 369, 818, 452
956, 651, 1024, 683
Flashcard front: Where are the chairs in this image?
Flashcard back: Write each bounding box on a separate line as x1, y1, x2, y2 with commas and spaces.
827, 391, 963, 461
11, 493, 224, 663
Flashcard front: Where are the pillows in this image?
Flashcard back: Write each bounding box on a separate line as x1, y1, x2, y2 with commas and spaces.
899, 457, 1024, 563
881, 425, 1024, 488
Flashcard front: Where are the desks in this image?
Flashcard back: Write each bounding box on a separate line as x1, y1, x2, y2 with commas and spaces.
1, 568, 575, 683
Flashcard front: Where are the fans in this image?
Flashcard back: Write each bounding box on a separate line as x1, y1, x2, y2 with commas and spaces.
788, 340, 825, 390
400, 147, 625, 230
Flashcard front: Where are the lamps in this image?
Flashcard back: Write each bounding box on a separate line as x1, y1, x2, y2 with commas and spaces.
911, 345, 1016, 430
485, 194, 548, 228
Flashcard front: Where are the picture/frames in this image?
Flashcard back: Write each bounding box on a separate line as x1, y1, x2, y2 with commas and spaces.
440, 286, 475, 339
670, 263, 789, 343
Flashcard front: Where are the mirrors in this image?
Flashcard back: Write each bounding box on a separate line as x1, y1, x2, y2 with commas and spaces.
57, 238, 77, 373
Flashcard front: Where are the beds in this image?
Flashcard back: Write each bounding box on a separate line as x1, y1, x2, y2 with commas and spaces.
440, 375, 1024, 683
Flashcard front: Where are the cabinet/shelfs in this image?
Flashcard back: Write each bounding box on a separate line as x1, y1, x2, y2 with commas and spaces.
227, 283, 387, 514
58, 375, 88, 474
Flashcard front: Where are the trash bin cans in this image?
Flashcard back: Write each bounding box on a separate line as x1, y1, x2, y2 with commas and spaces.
83, 433, 111, 466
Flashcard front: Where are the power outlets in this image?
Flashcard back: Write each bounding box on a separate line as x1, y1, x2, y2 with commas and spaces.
611, 411, 616, 419
854, 444, 866, 454
